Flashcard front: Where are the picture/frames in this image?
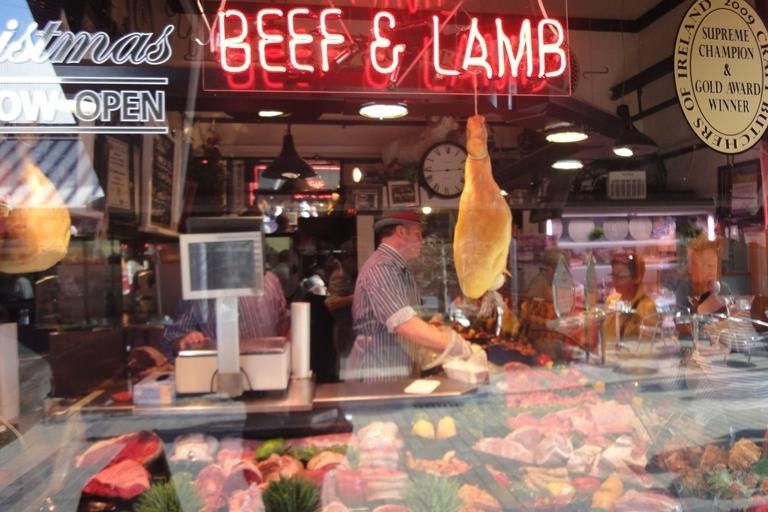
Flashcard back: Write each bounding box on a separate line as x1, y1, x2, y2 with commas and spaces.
348, 184, 383, 215
387, 179, 420, 208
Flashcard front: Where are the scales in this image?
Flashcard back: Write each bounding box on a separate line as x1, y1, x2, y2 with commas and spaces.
176, 230, 291, 394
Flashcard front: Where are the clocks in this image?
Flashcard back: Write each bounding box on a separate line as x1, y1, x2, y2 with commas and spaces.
418, 141, 468, 200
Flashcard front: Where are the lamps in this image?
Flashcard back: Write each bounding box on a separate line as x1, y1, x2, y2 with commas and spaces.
611, 2, 661, 156
356, 98, 410, 123
261, 124, 319, 182
544, 118, 589, 143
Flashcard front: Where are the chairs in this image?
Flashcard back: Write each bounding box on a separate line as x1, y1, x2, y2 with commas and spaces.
635, 310, 678, 352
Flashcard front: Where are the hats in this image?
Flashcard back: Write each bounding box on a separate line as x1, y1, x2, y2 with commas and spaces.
371, 207, 430, 231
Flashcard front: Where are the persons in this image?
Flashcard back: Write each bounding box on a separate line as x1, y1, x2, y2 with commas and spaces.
341, 212, 473, 461
604, 252, 659, 387
121, 246, 150, 326
159, 238, 356, 384
698, 279, 726, 311
4, 275, 35, 326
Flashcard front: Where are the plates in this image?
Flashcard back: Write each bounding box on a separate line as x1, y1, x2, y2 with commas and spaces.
547, 218, 654, 242
